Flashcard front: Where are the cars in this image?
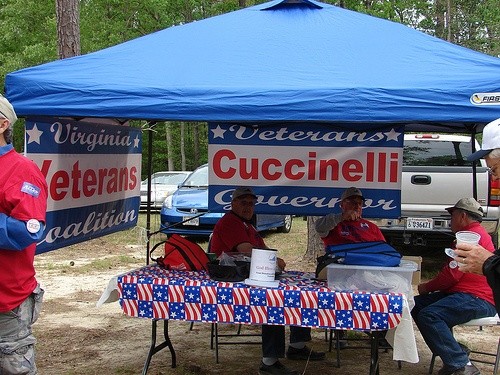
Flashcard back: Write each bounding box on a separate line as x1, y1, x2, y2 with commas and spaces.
159, 163, 293, 239
139, 171, 194, 213
362, 133, 500, 249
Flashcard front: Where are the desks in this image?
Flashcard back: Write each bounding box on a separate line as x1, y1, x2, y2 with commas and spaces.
96, 264, 419, 375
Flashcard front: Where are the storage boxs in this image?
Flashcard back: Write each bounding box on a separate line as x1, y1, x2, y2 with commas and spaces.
326, 260, 417, 292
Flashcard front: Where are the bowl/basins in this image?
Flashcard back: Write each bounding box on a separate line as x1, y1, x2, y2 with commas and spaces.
223, 253, 252, 279
207, 260, 245, 282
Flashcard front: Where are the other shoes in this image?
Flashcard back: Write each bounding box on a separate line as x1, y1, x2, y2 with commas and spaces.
452, 361, 480, 375
438, 341, 472, 375
333, 333, 350, 350
286, 344, 326, 361
257, 359, 297, 375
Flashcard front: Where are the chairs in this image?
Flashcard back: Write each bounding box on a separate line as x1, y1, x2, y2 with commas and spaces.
429, 314, 500, 375
207, 232, 262, 362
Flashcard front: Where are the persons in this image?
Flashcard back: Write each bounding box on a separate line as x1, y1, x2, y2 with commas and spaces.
454, 118, 500, 316
314, 186, 386, 250
209, 189, 326, 375
412, 196, 495, 375
0, 93, 49, 375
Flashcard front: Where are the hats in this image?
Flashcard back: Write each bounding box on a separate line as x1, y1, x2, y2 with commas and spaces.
445, 197, 484, 218
0, 94, 18, 127
231, 187, 258, 203
466, 118, 500, 162
341, 186, 365, 202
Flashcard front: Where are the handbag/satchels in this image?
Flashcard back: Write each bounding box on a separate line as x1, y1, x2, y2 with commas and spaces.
315, 239, 402, 278
150, 233, 211, 273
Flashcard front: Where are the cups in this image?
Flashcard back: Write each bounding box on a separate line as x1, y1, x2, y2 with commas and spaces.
455, 231, 481, 268
207, 252, 216, 262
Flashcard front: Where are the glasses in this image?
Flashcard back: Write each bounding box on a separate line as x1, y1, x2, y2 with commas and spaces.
486, 162, 500, 176
240, 199, 256, 207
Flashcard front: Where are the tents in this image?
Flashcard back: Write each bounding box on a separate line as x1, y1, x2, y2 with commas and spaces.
3, 0, 500, 265
